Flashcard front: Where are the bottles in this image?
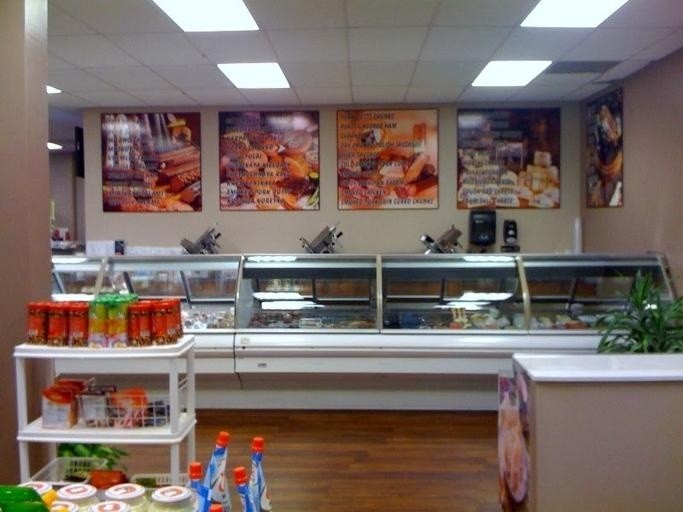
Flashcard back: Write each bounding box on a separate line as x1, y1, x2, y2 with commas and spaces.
184, 430, 272, 511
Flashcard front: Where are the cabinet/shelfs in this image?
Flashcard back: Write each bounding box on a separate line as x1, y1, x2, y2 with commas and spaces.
11, 332, 197, 485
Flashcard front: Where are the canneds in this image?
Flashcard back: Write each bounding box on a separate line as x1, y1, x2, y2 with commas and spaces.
26, 296, 183, 348
17, 482, 197, 512
